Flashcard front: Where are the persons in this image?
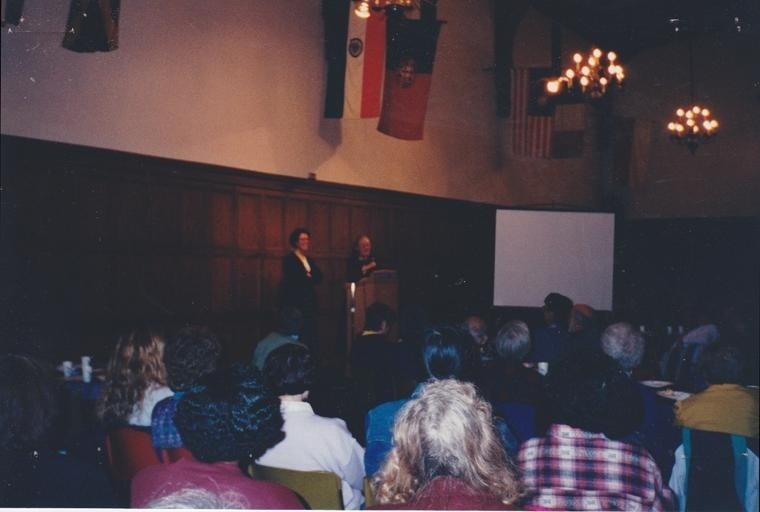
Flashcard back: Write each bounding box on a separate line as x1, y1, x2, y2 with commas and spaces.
1, 291, 760, 511
346, 235, 386, 283
278, 228, 321, 342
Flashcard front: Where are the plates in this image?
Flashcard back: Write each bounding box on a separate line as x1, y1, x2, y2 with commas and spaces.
641, 380, 674, 388
656, 390, 693, 401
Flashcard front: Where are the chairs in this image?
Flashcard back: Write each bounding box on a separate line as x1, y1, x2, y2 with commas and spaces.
58, 398, 760, 510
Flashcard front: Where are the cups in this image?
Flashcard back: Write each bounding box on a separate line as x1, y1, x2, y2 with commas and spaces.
80, 356, 92, 383
538, 361, 549, 376
63, 361, 72, 378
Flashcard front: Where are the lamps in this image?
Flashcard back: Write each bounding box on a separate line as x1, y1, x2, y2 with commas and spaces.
545, 48, 627, 100
666, 1, 721, 156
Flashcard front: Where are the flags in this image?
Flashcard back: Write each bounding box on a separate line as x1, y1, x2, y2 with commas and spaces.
60, 0, 121, 54
324, 0, 386, 120
377, 15, 441, 142
509, 66, 551, 161
